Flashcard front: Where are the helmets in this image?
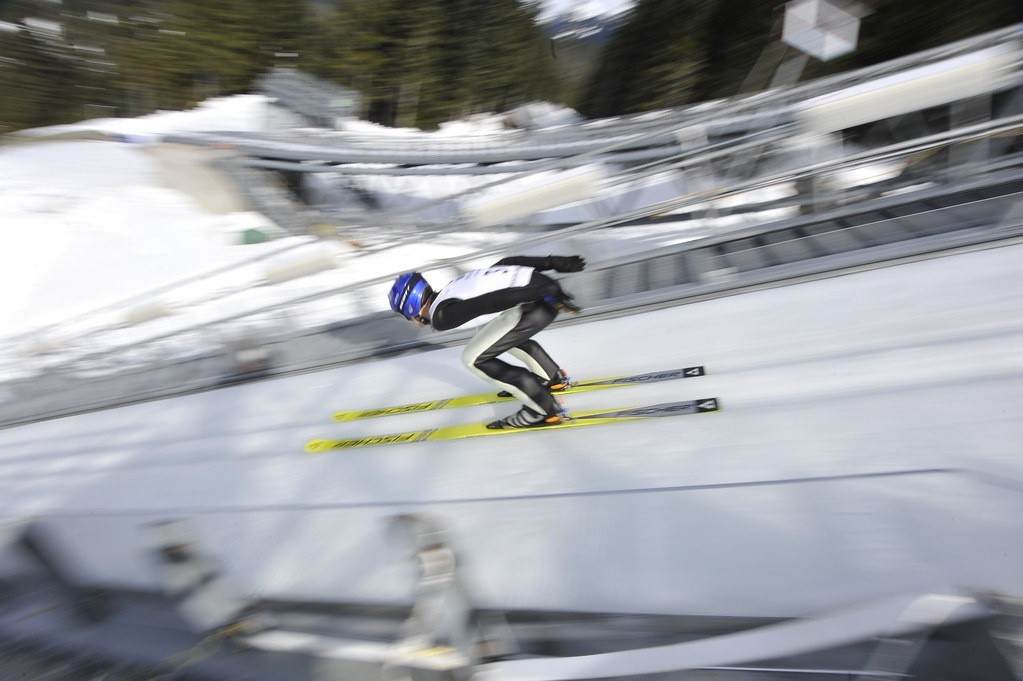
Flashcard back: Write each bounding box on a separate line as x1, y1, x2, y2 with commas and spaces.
388, 273, 432, 317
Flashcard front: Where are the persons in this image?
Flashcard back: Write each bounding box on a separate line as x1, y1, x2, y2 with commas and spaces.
387, 254, 586, 427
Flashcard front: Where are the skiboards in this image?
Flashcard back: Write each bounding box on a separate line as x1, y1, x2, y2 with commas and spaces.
305, 364, 720, 454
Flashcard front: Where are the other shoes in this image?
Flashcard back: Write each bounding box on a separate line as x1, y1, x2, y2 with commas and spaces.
550, 373, 569, 393
505, 405, 555, 428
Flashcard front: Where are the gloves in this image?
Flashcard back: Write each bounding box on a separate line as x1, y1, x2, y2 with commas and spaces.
542, 287, 569, 310
550, 255, 584, 273
